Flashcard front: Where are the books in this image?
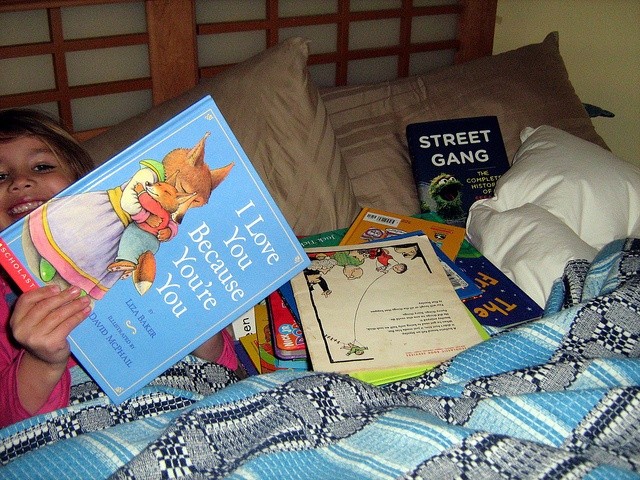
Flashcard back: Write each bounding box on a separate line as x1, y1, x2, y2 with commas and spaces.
0, 95, 313, 406
232, 208, 544, 384
405, 114, 511, 229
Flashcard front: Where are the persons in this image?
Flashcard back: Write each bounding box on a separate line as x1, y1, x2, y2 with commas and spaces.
393, 243, 421, 260
330, 250, 365, 280
0, 108, 237, 429
369, 248, 407, 273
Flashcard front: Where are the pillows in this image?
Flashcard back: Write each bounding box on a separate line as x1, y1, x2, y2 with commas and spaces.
463, 125, 640, 308
76, 33, 362, 238
317, 29, 612, 219
